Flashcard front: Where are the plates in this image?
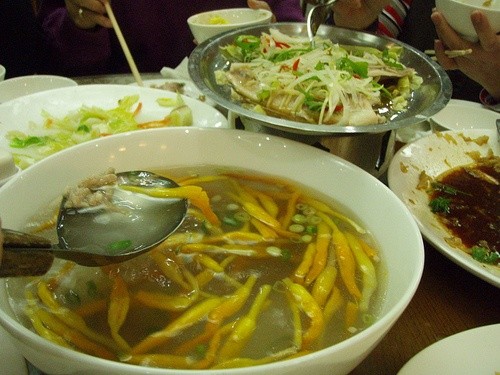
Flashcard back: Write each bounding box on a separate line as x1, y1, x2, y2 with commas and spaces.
126, 78, 218, 108
386, 127, 500, 286
396, 323, 499, 375
0, 85, 228, 187
395, 98, 500, 142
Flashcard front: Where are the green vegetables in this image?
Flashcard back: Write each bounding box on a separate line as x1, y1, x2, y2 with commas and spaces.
426, 181, 500, 266
233, 34, 406, 113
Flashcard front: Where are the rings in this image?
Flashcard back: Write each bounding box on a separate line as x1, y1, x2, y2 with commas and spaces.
78, 8, 83, 17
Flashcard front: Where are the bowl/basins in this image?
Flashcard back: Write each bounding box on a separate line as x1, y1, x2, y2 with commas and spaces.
0, 74, 77, 107
0, 127, 425, 375
187, 8, 273, 45
434, 0, 500, 43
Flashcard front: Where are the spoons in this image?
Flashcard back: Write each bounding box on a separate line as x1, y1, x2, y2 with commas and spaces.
0, 170, 188, 276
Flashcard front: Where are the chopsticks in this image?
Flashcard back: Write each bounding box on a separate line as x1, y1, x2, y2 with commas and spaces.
424, 49, 472, 61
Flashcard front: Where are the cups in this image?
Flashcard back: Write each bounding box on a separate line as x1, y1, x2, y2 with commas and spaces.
0, 65, 6, 82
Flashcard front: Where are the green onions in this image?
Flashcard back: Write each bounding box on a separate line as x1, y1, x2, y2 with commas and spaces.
80, 193, 323, 355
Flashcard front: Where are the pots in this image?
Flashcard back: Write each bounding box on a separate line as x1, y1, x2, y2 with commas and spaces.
187, 21, 452, 137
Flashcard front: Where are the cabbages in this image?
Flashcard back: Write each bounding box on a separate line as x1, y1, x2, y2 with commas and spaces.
6, 91, 190, 173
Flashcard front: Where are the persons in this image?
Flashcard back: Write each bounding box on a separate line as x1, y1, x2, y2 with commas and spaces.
0, 0, 306, 76
331, 0, 500, 115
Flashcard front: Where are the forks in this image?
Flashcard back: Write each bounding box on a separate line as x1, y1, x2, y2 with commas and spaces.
306, 0, 339, 42
